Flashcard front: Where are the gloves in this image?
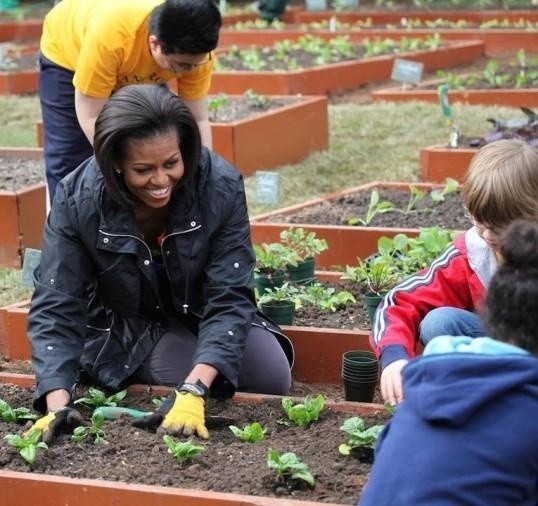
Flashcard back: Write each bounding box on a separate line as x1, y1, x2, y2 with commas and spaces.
129, 377, 214, 441
19, 405, 87, 449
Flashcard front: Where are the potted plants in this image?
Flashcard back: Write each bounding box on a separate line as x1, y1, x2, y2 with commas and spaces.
251, 243, 305, 299
278, 225, 328, 286
358, 260, 401, 322
260, 280, 296, 326
358, 233, 416, 281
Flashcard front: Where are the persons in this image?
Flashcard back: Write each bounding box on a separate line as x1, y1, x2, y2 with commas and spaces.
39, 0, 223, 207
360, 255, 538, 504
369, 138, 537, 406
21, 83, 298, 444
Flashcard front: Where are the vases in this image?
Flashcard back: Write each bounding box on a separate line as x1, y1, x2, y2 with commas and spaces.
341, 350, 379, 402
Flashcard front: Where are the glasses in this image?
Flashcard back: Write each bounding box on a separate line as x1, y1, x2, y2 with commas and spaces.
462, 214, 511, 237
165, 49, 216, 75
